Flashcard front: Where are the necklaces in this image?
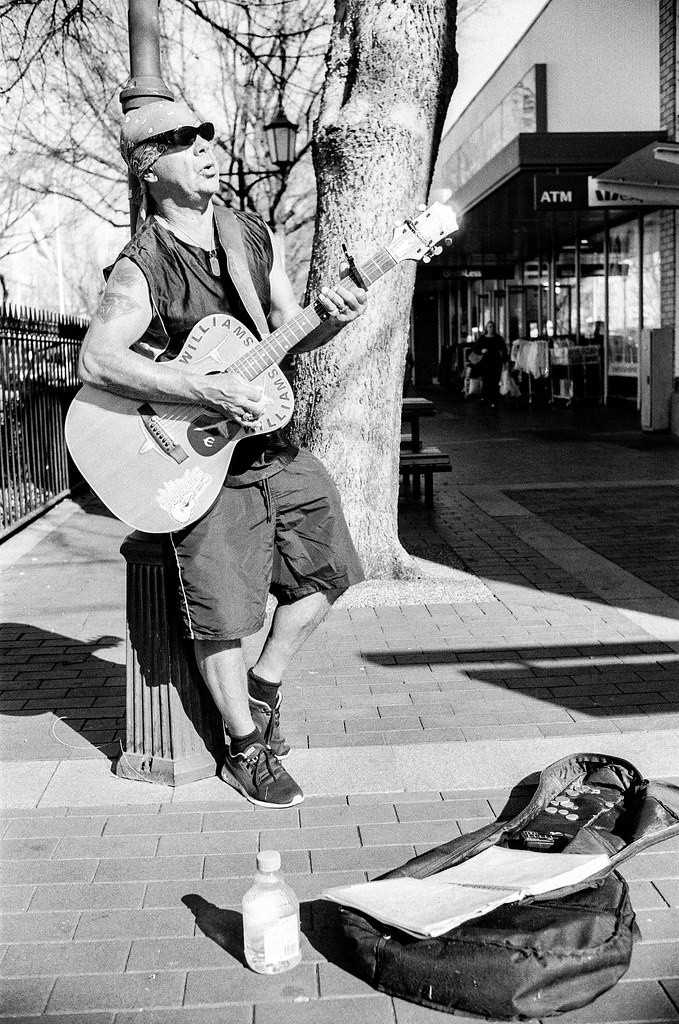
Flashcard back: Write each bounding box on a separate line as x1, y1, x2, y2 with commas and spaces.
155, 208, 221, 277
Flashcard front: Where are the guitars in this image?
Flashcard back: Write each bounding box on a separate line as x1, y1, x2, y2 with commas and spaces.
64, 199, 461, 534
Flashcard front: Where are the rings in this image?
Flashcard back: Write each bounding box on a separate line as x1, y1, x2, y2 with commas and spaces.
339, 305, 349, 316
241, 410, 254, 424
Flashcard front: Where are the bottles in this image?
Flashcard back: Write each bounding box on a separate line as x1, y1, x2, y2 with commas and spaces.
241, 850, 302, 975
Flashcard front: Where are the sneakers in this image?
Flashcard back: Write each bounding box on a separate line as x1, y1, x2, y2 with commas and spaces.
221, 738, 303, 808
247, 690, 292, 758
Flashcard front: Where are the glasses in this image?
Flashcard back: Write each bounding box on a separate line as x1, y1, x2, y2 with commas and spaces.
126, 122, 214, 155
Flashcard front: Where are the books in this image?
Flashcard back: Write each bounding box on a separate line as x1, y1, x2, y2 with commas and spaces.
322, 845, 611, 939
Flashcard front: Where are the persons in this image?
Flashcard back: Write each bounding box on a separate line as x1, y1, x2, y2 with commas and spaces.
472, 322, 508, 408
79, 102, 372, 808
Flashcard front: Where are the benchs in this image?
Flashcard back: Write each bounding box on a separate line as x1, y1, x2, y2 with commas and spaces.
400, 434, 451, 510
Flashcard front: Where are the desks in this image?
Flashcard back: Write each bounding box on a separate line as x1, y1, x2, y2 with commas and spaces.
402, 398, 435, 454
